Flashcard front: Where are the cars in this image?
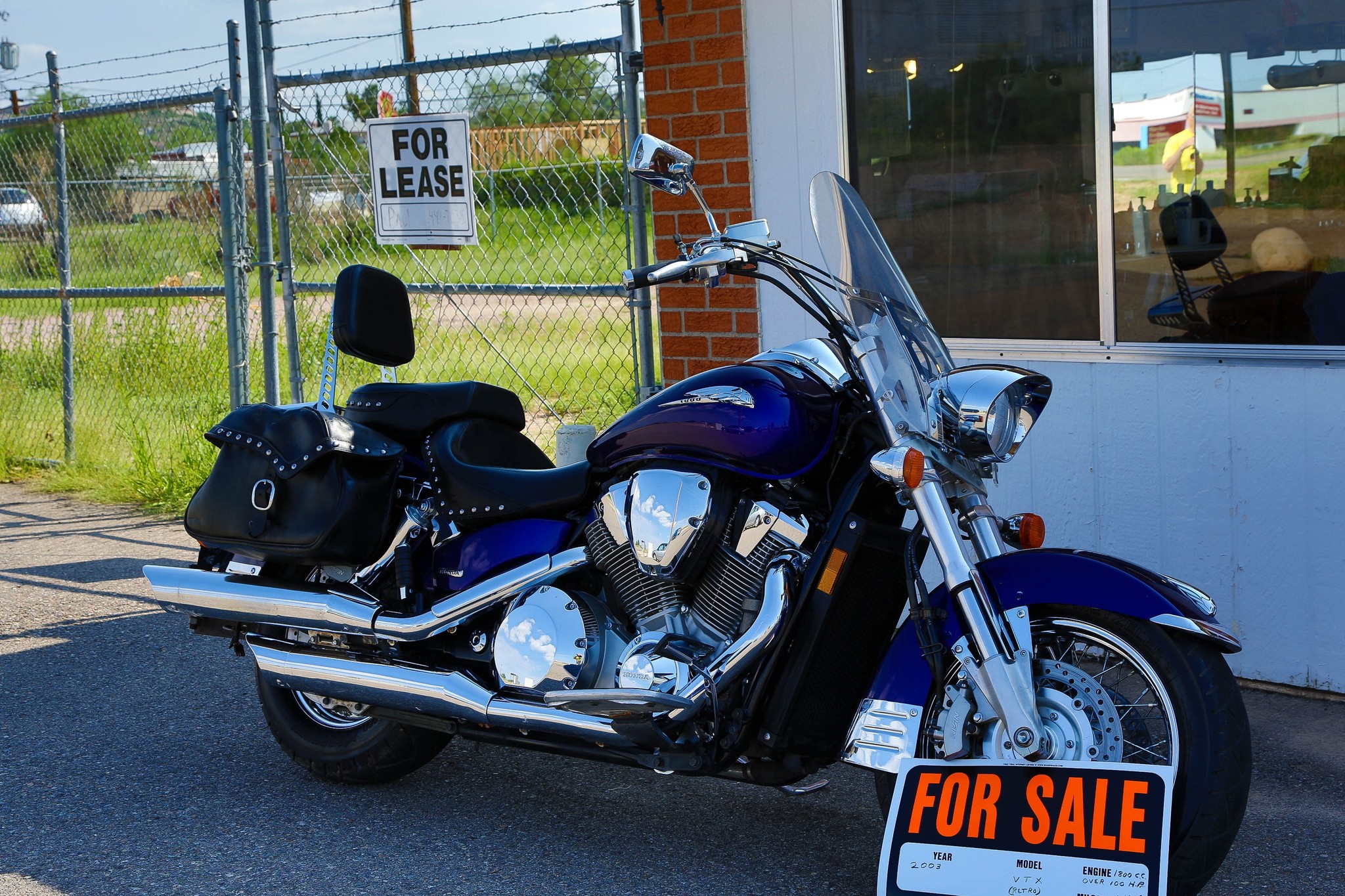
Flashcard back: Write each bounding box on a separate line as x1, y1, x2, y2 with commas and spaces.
1, 188, 48, 232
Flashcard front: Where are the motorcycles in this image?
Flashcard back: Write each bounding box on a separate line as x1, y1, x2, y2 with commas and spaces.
142, 134, 1247, 896
1144, 194, 1344, 345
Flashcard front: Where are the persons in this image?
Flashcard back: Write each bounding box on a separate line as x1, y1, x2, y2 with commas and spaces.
1252, 227, 1345, 346
1162, 101, 1204, 195
